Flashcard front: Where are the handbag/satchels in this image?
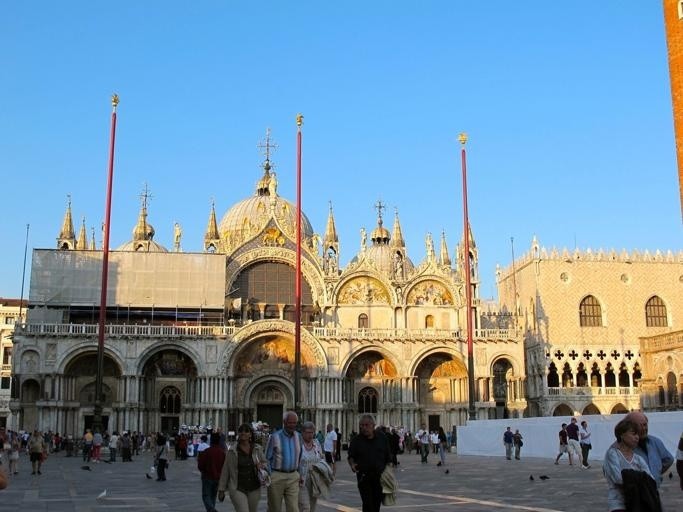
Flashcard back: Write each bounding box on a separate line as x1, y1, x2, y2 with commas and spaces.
258, 468, 272, 489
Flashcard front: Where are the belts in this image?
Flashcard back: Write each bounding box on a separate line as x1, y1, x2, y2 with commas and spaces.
271, 468, 297, 473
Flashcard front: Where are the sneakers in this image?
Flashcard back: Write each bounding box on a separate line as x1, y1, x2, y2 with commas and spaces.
7, 447, 187, 483
399, 449, 448, 467
505, 454, 590, 472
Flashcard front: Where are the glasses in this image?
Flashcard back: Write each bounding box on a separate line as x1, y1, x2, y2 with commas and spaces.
238, 429, 250, 433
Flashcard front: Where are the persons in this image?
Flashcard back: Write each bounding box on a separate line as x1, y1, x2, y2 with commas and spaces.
513, 429, 523, 460
555, 424, 571, 464
504, 427, 514, 460
350, 424, 448, 466
624, 412, 674, 503
676, 432, 683, 491
580, 421, 592, 470
198, 411, 343, 511
347, 414, 394, 512
565, 417, 583, 466
604, 420, 655, 512
1, 423, 187, 489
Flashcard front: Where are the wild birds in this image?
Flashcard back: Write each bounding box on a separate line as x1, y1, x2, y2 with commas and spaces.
397, 461, 400, 464
436, 461, 442, 467
669, 471, 673, 480
539, 475, 550, 480
145, 472, 153, 480
529, 474, 534, 480
80, 465, 92, 472
445, 469, 449, 474
95, 487, 107, 503
